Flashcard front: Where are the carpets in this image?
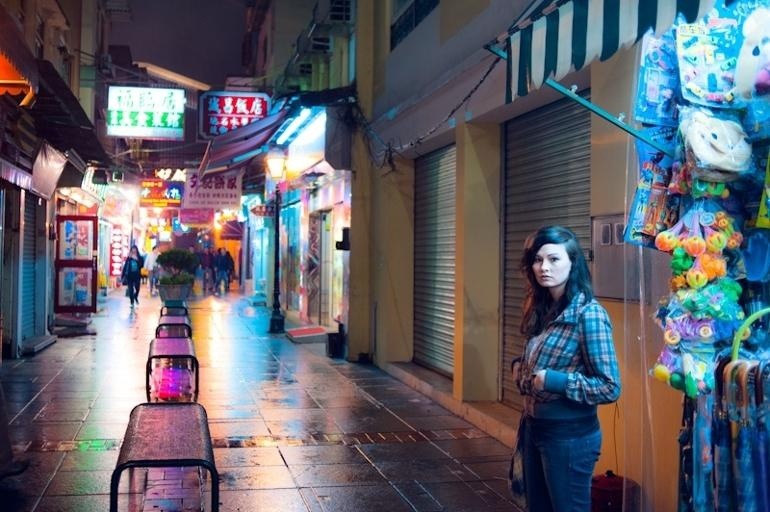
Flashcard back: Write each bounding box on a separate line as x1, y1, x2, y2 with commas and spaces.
288, 327, 327, 337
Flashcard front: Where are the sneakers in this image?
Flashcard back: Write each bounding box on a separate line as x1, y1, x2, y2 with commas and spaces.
130, 299, 138, 308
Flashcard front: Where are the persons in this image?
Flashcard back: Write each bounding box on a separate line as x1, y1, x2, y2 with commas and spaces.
145, 245, 161, 296
187, 246, 235, 297
511, 226, 621, 511
125, 246, 144, 306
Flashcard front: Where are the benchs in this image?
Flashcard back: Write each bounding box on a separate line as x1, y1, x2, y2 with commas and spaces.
145, 298, 201, 403
109, 401, 221, 512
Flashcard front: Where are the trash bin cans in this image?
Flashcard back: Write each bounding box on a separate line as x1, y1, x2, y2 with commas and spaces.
326, 333, 344, 359
591, 473, 641, 512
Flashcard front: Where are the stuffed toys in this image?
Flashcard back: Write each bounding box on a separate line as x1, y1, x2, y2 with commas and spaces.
639, 36, 752, 399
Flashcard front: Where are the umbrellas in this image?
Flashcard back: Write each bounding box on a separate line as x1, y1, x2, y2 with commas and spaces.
678, 355, 770, 512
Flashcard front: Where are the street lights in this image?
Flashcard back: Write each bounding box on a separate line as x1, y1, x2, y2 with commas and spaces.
259, 149, 288, 339
